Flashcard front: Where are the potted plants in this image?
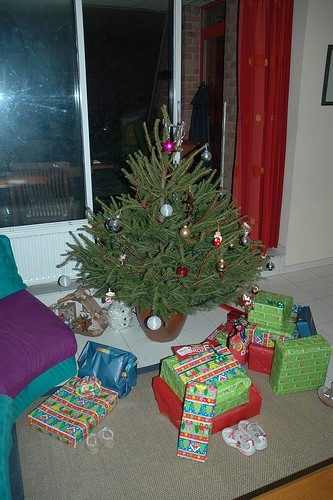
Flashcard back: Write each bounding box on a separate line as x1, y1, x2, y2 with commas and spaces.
59, 106, 278, 341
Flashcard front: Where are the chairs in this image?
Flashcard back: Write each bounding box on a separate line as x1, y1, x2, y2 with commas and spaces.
7, 161, 77, 224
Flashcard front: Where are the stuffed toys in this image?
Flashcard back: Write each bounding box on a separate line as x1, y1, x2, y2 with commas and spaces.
106, 301, 132, 330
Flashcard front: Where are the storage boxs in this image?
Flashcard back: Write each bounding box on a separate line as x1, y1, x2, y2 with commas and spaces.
152, 289, 331, 462
28, 379, 119, 449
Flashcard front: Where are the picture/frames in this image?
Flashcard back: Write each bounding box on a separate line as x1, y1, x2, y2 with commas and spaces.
321, 45, 333, 106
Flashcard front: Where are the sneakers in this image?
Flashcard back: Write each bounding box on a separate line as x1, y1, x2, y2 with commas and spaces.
238, 420, 267, 450
221, 428, 256, 456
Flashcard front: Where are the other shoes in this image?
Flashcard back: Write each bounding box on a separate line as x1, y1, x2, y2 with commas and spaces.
318, 386, 333, 408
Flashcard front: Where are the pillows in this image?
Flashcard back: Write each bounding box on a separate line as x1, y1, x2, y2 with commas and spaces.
1, 290, 77, 399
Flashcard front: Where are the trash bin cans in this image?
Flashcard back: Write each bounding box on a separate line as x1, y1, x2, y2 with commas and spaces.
120, 118, 148, 162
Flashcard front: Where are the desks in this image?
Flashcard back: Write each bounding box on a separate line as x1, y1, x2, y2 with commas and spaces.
0, 160, 117, 201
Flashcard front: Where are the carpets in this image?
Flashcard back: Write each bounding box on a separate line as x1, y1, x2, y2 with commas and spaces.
9, 354, 333, 500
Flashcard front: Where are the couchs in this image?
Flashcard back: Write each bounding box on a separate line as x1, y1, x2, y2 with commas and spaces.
0, 233, 79, 498
